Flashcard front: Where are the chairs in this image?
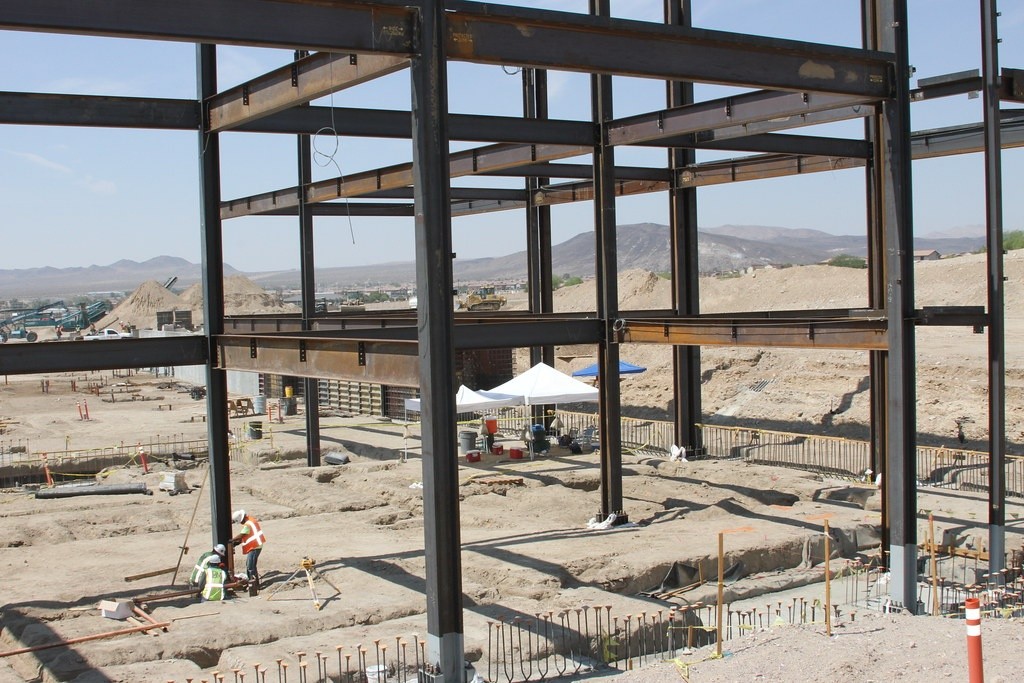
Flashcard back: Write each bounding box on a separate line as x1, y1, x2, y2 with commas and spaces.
569, 427, 595, 448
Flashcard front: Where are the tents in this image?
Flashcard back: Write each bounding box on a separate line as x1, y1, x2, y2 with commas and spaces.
405, 361, 647, 460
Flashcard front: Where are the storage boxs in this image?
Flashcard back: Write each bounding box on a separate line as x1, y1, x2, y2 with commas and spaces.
492, 445, 503, 454
466, 452, 480, 462
97, 597, 133, 619
510, 449, 523, 458
160, 471, 185, 491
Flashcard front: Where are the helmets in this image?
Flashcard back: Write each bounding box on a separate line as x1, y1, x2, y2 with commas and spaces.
233, 510, 245, 523
209, 555, 221, 563
214, 544, 225, 556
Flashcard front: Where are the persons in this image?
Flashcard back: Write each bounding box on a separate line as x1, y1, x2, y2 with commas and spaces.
189, 510, 266, 603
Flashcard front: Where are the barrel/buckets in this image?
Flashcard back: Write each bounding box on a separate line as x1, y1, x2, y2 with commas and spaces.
249, 421, 263, 439
458, 430, 477, 454
254, 396, 266, 415
285, 386, 293, 397
291, 398, 298, 415
366, 665, 387, 683
485, 417, 498, 432
279, 398, 290, 415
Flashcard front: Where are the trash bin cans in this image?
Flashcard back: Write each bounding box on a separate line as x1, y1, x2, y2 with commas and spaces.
254, 396, 266, 414
459, 430, 478, 454
249, 421, 262, 439
285, 387, 293, 397
280, 397, 297, 416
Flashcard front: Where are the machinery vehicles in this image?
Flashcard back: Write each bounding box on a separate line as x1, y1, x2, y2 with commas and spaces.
0, 298, 68, 343
456, 283, 507, 312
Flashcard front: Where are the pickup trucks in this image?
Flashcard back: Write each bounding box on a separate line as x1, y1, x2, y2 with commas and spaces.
83, 329, 132, 340
